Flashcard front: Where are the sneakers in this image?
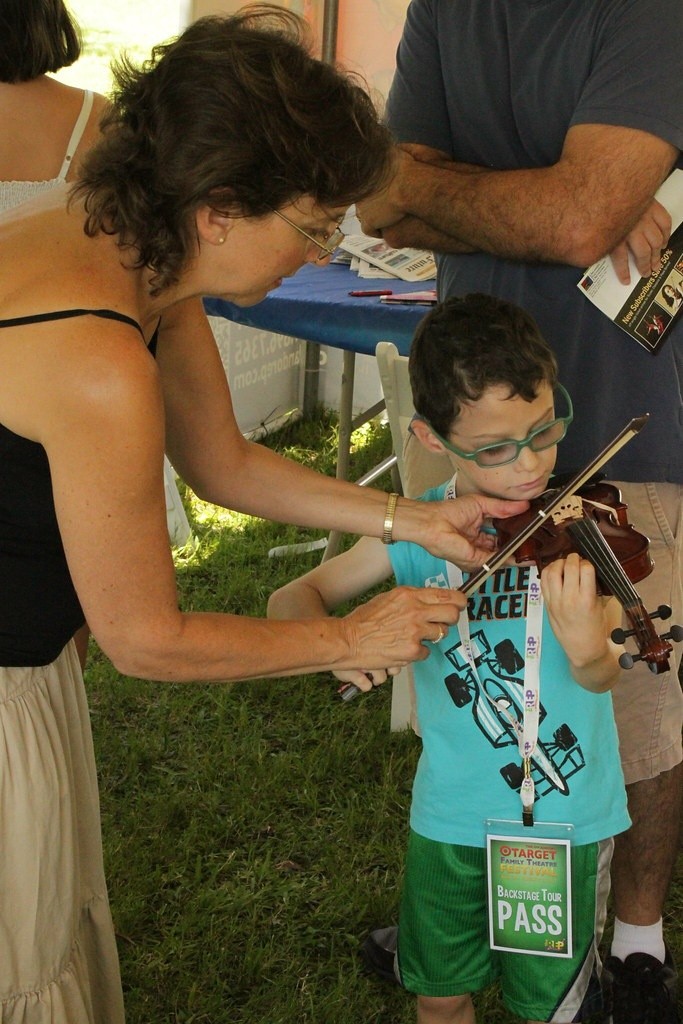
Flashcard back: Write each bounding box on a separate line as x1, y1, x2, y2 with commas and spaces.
368, 926, 401, 985
602, 938, 682, 1024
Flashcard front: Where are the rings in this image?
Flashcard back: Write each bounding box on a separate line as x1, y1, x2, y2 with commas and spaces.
431, 623, 445, 645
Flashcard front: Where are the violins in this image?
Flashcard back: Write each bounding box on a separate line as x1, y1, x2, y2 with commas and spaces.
490, 483, 683, 676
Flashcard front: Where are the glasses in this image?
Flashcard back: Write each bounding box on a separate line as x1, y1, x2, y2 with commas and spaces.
426, 378, 573, 468
273, 208, 346, 260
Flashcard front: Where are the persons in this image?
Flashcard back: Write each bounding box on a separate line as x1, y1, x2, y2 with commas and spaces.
0, 0, 114, 222
266, 292, 633, 1024
353, 0, 683, 1024
0, 0, 536, 1024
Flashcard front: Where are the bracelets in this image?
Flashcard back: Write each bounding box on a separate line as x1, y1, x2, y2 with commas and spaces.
382, 493, 400, 544
